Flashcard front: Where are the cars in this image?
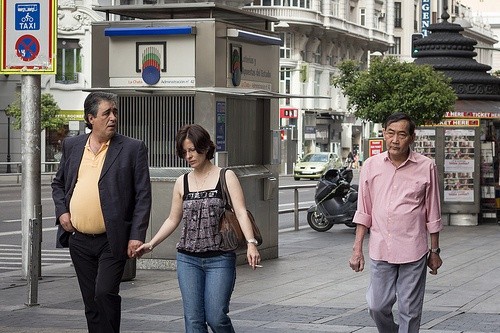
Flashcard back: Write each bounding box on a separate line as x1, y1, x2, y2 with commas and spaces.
292, 151, 343, 181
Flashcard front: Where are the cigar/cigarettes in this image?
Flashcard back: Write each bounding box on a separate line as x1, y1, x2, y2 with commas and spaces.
252, 265, 263, 268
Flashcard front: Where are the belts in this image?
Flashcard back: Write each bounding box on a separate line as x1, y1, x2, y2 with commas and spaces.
75, 230, 107, 238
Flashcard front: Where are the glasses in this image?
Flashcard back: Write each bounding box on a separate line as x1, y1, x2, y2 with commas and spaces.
184, 147, 197, 156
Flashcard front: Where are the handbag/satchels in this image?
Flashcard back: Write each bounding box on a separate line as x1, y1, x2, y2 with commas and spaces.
218, 167, 263, 252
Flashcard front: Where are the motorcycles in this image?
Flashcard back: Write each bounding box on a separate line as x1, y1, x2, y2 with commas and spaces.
307, 166, 372, 233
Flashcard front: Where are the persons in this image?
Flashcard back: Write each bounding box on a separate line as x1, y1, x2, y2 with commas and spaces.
50, 91, 152, 333
412, 133, 474, 191
135, 123, 261, 333
348, 112, 443, 333
347, 152, 359, 169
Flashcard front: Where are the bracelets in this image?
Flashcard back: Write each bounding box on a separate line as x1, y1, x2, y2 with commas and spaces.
245, 239, 258, 246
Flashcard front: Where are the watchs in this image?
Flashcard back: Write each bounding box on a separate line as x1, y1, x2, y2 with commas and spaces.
429, 248, 440, 254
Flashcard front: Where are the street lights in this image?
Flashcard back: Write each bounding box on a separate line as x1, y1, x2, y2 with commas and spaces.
363, 50, 382, 139
4, 106, 15, 171
